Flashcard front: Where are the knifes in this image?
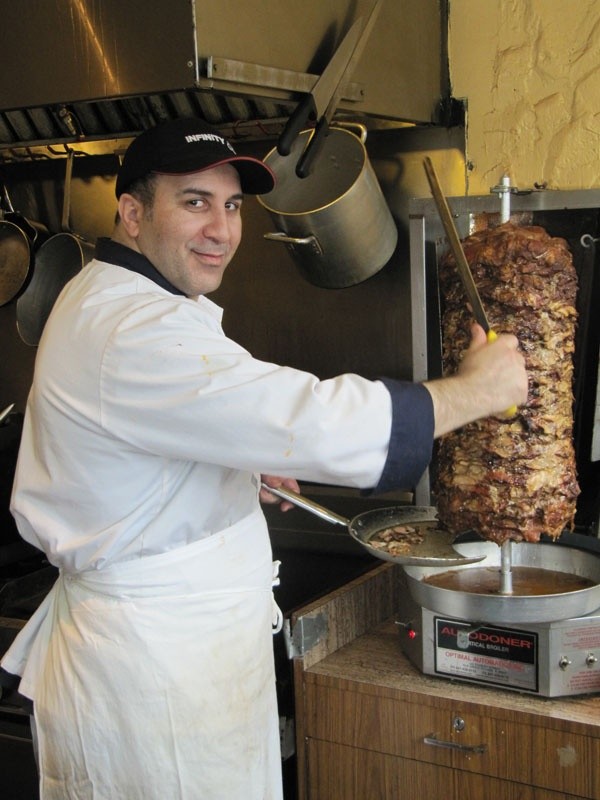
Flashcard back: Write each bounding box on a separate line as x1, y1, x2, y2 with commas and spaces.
294, 0, 383, 178
420, 155, 517, 423
275, 17, 364, 156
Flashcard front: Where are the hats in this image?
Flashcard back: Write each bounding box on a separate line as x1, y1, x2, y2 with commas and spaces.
114, 116, 276, 201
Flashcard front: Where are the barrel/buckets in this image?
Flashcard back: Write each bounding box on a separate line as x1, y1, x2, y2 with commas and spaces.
255, 120, 400, 290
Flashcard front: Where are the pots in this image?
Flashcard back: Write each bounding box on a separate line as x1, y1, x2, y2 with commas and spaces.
0, 150, 99, 347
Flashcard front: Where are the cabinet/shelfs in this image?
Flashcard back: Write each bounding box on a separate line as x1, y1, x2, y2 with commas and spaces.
294, 675, 600, 800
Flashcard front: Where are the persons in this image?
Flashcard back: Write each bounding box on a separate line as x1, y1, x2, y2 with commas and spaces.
0, 121, 527, 800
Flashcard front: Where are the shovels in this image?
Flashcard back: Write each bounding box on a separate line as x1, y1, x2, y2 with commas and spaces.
262, 483, 487, 567
